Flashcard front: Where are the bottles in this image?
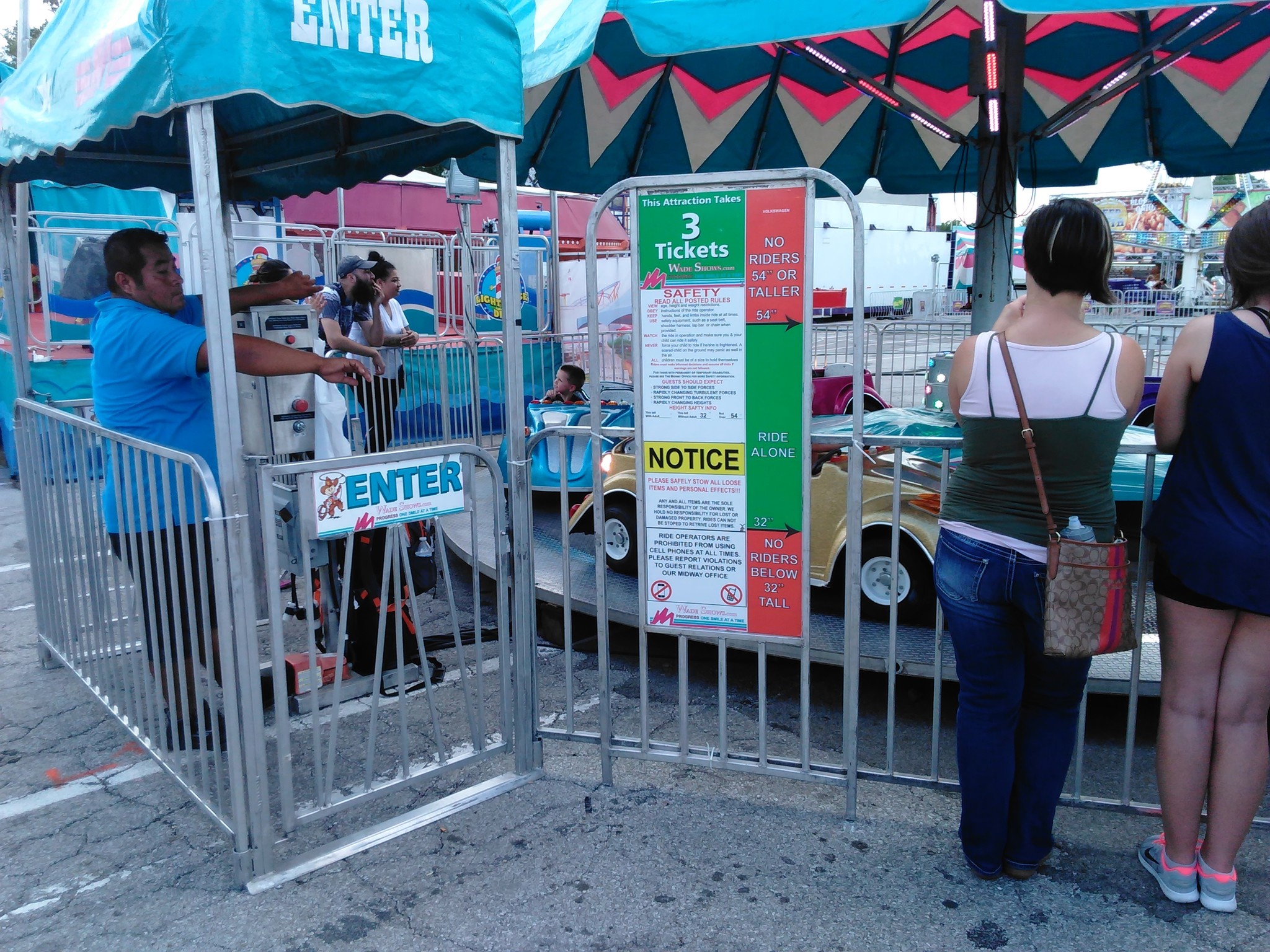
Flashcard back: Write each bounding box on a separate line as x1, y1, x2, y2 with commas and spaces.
1060, 515, 1098, 544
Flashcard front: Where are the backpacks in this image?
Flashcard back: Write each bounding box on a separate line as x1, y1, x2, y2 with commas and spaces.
311, 518, 440, 679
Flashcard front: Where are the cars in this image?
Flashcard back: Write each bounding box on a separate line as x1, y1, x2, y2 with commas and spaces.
493, 364, 958, 627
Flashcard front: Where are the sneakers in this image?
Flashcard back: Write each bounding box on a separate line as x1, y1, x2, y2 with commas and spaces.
1196, 835, 1238, 912
1137, 833, 1200, 902
165, 707, 227, 752
261, 676, 294, 709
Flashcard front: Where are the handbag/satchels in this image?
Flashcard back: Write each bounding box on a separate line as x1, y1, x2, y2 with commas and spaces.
1042, 530, 1140, 660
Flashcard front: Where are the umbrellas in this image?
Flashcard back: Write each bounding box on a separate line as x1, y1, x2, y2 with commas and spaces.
449, 0, 1270, 335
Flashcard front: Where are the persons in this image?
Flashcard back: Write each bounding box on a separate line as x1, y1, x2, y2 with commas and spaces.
249, 257, 327, 324
1145, 275, 1156, 287
542, 364, 590, 402
928, 193, 1150, 878
344, 251, 420, 454
311, 254, 387, 375
1136, 201, 1269, 912
1152, 277, 1167, 291
86, 227, 376, 755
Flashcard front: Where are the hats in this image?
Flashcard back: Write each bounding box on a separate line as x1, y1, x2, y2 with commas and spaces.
337, 255, 379, 278
